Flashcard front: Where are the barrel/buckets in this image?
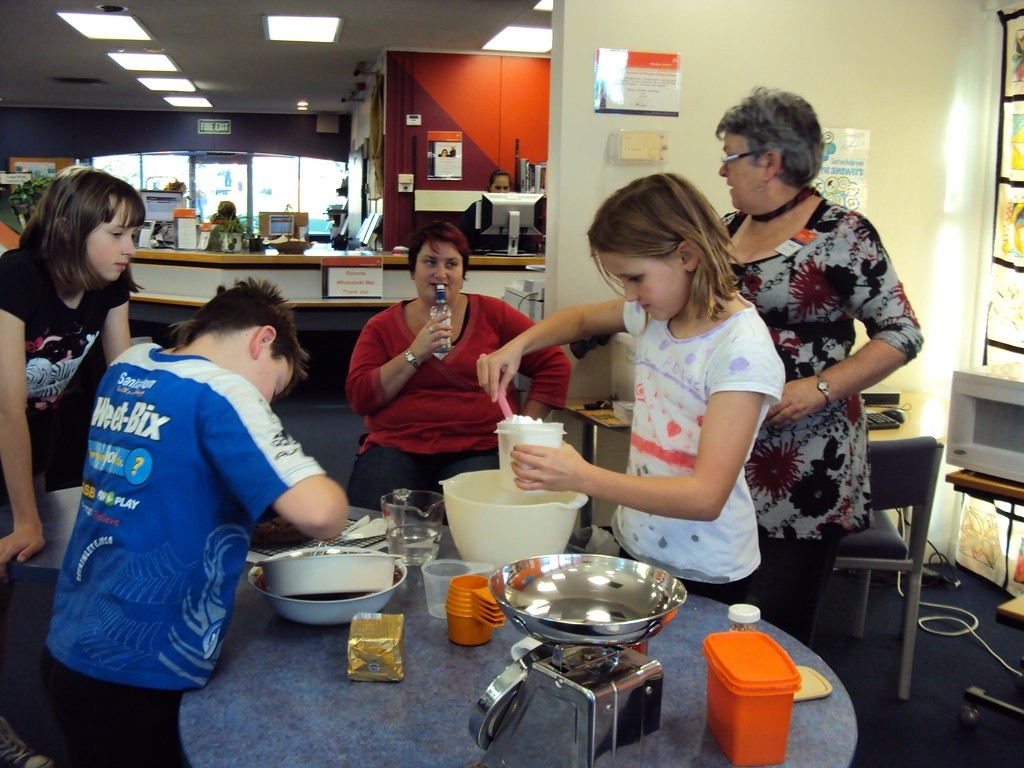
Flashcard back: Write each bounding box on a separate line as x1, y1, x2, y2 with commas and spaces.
494, 422, 567, 494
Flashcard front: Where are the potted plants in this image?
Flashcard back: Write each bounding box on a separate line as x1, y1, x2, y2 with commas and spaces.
208, 208, 253, 252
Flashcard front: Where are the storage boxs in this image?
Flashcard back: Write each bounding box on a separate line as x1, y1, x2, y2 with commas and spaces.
702, 628, 800, 767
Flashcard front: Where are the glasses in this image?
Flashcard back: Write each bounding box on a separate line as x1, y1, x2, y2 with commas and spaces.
721, 148, 785, 170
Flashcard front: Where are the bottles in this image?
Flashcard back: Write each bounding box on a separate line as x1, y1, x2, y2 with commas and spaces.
430, 284, 451, 352
726, 605, 762, 633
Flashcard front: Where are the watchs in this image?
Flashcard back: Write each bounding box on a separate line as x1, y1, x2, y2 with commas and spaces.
404, 348, 420, 370
812, 374, 833, 408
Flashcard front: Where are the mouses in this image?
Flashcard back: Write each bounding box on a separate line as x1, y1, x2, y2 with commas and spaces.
881, 408, 905, 424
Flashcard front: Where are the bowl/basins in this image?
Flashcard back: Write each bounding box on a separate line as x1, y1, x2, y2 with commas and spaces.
248, 546, 407, 625
438, 469, 589, 578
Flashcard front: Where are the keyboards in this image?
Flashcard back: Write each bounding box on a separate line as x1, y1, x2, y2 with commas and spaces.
866, 412, 900, 430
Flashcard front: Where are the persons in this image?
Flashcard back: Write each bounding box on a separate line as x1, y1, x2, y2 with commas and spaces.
343, 219, 572, 531
0, 168, 144, 768
41, 273, 351, 768
456, 170, 533, 253
714, 89, 924, 646
477, 175, 786, 616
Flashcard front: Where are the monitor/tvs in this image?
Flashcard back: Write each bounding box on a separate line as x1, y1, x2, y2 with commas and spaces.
268, 215, 294, 238
481, 193, 545, 256
140, 190, 183, 225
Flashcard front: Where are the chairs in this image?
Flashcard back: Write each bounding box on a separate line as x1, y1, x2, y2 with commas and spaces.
820, 434, 948, 702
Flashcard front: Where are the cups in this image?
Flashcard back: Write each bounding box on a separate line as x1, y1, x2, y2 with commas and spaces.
380, 488, 444, 567
421, 561, 495, 619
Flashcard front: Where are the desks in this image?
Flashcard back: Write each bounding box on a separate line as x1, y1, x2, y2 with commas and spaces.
0, 485, 415, 585
561, 391, 949, 538
945, 469, 1024, 723
180, 526, 858, 768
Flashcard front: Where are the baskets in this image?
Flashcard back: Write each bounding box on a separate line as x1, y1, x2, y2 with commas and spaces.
269, 205, 315, 254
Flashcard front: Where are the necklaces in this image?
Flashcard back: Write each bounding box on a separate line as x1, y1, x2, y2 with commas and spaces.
752, 186, 812, 225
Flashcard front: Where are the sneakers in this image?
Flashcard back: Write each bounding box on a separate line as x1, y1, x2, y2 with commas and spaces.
0, 717, 54, 768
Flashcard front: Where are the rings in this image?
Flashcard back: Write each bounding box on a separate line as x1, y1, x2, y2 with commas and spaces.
428, 326, 435, 332
